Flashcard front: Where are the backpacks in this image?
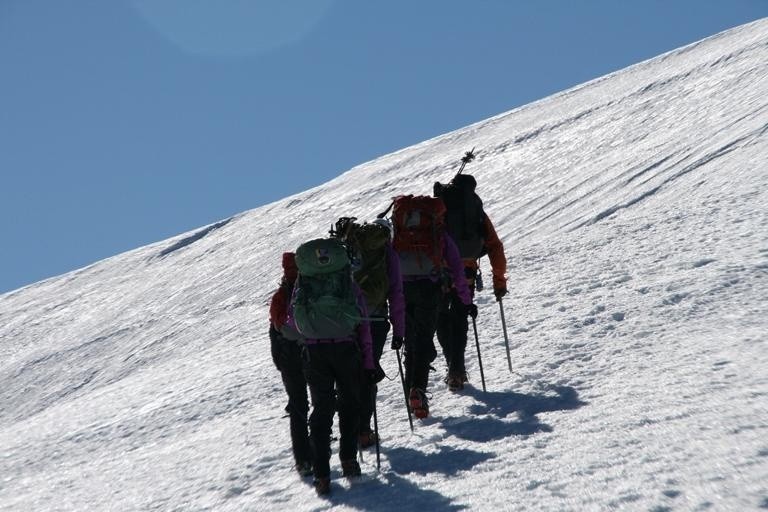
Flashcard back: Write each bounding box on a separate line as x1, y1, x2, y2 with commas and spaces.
390, 193, 448, 256
291, 237, 362, 341
347, 223, 391, 314
432, 181, 484, 258
268, 251, 318, 347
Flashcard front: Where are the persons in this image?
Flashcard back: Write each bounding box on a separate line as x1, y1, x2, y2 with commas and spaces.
338, 217, 407, 451
267, 262, 325, 478
287, 259, 380, 494
430, 173, 508, 392
385, 223, 478, 422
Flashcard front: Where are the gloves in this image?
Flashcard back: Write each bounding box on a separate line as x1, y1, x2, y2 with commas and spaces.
390, 335, 402, 349
463, 304, 478, 319
493, 287, 508, 302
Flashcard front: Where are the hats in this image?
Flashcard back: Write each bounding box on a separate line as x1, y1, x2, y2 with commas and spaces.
453, 174, 477, 191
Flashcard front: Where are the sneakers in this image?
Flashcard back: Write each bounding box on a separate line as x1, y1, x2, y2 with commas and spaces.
444, 372, 469, 391
357, 430, 381, 450
341, 460, 362, 476
296, 460, 330, 494
406, 385, 430, 419
329, 435, 339, 444
